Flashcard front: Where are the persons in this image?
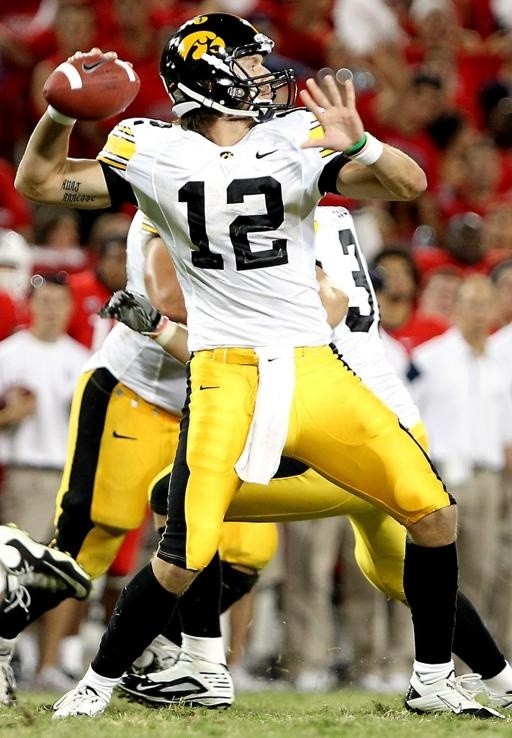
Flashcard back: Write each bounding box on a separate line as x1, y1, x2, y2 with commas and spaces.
0, 1, 512, 724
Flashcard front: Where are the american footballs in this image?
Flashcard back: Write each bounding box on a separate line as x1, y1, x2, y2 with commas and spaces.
43, 56, 140, 121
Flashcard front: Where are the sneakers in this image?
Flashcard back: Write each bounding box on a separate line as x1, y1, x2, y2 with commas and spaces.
118, 650, 237, 712
0, 645, 19, 705
124, 634, 181, 675
404, 667, 507, 722
0, 522, 97, 604
52, 679, 116, 722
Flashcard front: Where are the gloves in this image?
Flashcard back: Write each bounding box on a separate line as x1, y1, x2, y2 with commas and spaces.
98, 288, 162, 339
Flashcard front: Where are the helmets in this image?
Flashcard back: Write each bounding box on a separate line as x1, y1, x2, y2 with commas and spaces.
159, 12, 298, 124
